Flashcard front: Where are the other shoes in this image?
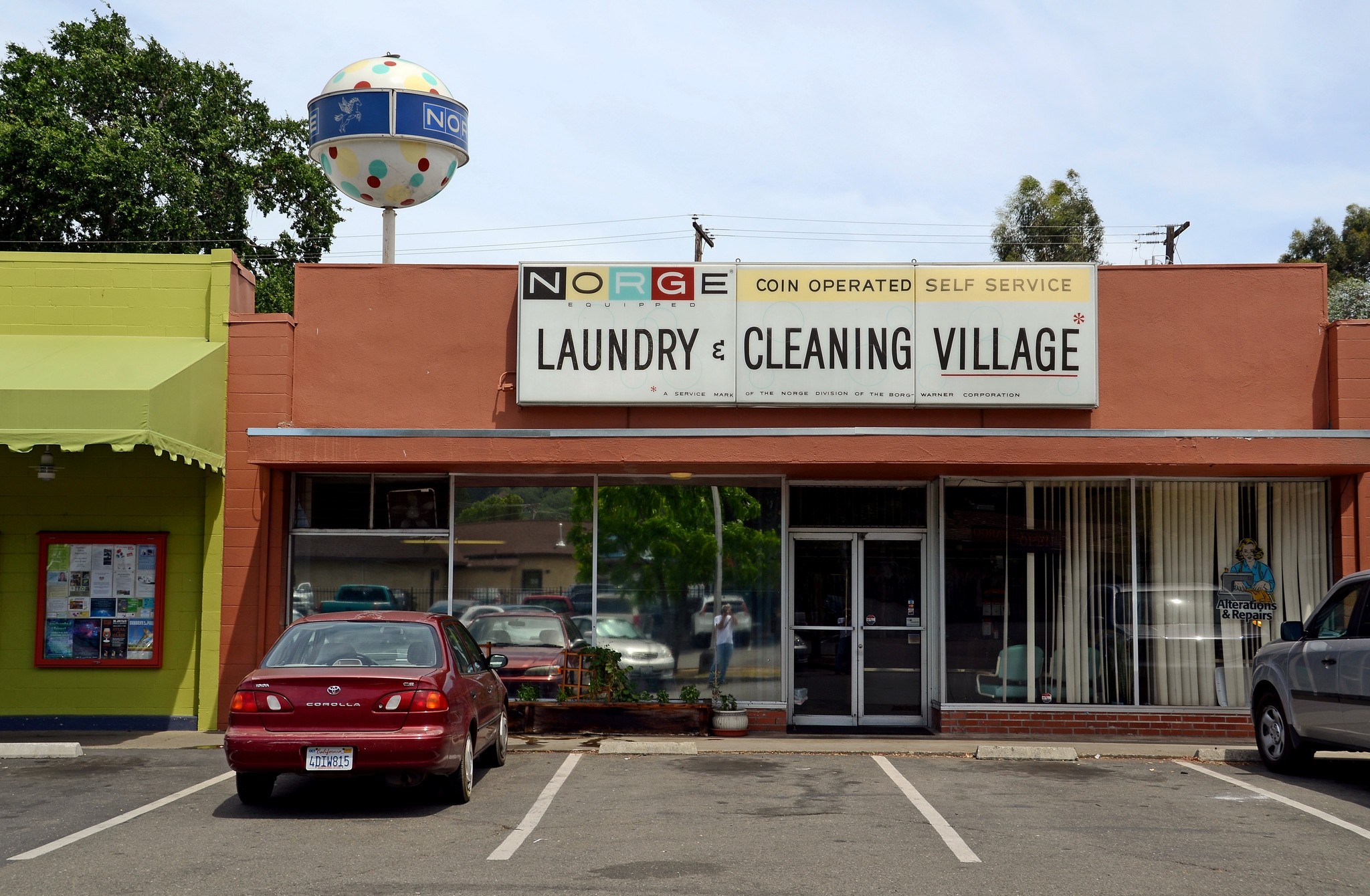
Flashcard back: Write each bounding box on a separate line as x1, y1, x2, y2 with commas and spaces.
707, 684, 719, 689
718, 680, 728, 685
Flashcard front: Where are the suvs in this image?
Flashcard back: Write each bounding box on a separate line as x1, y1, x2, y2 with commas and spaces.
691, 594, 751, 648
1250, 569, 1370, 775
1087, 583, 1270, 706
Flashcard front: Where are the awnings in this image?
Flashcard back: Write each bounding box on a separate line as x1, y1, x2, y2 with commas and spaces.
0, 336, 228, 478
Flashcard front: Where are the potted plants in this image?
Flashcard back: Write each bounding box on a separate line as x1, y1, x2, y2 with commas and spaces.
713, 693, 749, 730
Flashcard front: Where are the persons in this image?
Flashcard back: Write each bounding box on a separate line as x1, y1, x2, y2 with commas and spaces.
708, 604, 737, 689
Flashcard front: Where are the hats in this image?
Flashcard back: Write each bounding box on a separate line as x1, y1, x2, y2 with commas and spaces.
722, 603, 731, 609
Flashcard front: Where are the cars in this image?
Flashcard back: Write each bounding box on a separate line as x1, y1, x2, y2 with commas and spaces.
293, 583, 653, 635
224, 611, 508, 806
465, 610, 587, 700
569, 615, 675, 691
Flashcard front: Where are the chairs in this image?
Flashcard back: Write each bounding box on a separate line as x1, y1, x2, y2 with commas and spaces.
974, 644, 1044, 704
318, 643, 357, 665
407, 642, 435, 666
1046, 648, 1102, 704
